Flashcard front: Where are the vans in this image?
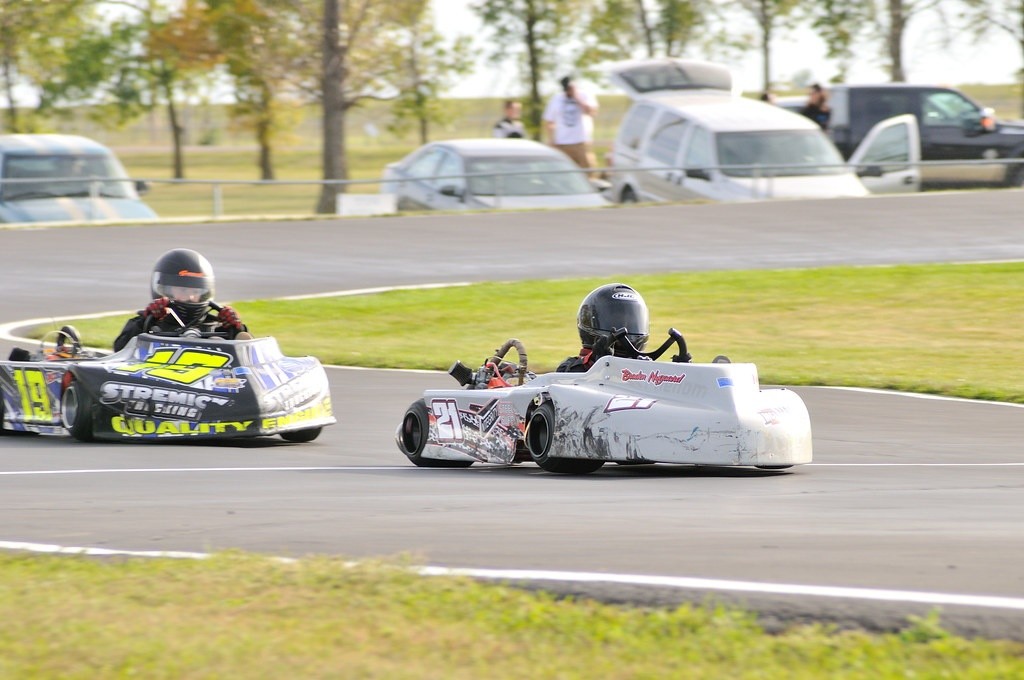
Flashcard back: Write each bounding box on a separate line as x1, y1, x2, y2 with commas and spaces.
597, 60, 921, 205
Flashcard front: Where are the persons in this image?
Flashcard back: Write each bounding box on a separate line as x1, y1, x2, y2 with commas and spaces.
556, 283, 650, 373
492, 99, 527, 138
800, 83, 831, 129
761, 92, 778, 106
542, 76, 600, 177
114, 247, 253, 354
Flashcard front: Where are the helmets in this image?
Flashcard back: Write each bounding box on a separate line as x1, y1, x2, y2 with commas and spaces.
150, 248, 215, 324
577, 283, 650, 358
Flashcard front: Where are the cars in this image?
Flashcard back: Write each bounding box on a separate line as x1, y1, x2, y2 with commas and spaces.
379, 138, 616, 212
0, 127, 160, 224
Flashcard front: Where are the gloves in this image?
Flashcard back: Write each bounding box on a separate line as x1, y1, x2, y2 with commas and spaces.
592, 335, 615, 355
144, 296, 169, 322
217, 306, 242, 329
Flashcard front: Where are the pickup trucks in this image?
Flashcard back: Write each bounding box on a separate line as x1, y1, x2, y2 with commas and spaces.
764, 81, 1024, 193
